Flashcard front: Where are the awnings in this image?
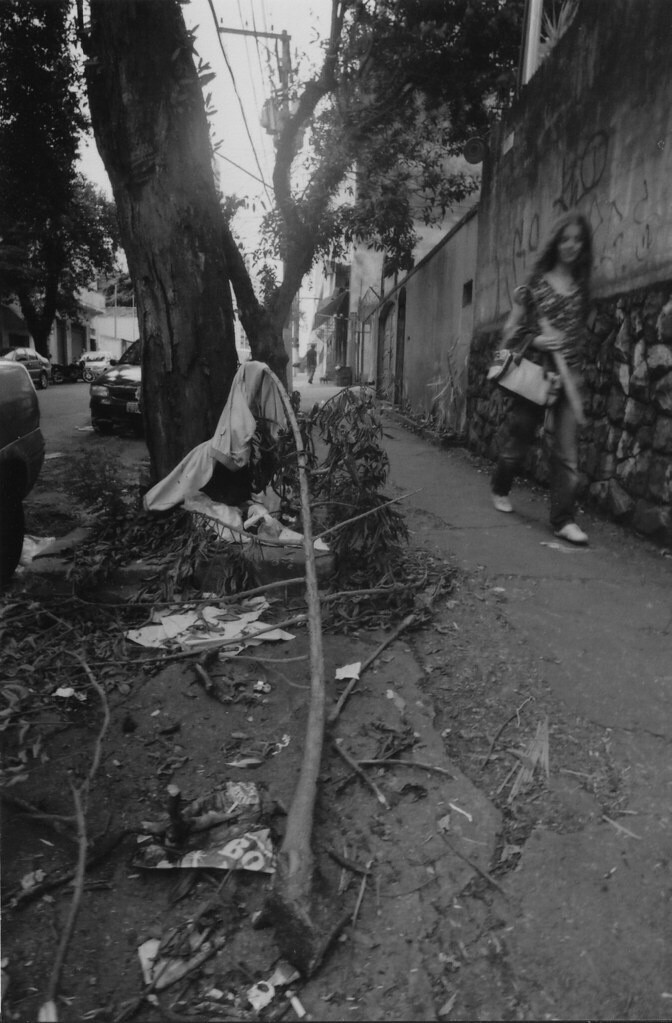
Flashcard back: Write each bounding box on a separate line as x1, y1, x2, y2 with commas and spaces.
312, 290, 349, 330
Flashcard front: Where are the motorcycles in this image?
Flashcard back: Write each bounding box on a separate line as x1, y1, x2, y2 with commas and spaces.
50, 358, 97, 384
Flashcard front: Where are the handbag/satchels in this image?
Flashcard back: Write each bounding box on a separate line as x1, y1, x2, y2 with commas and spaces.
487, 333, 555, 408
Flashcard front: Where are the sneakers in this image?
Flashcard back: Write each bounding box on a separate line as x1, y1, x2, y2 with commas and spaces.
554, 523, 588, 542
491, 492, 514, 513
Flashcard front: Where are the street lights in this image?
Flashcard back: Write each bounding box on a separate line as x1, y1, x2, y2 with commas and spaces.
257, 93, 306, 395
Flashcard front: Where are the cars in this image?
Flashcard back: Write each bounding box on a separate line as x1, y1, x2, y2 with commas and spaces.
76, 350, 117, 378
89, 338, 145, 437
0, 361, 47, 594
0, 346, 52, 389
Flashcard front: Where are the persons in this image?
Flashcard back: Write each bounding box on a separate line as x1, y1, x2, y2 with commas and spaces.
79, 347, 86, 360
306, 344, 317, 384
491, 208, 595, 541
